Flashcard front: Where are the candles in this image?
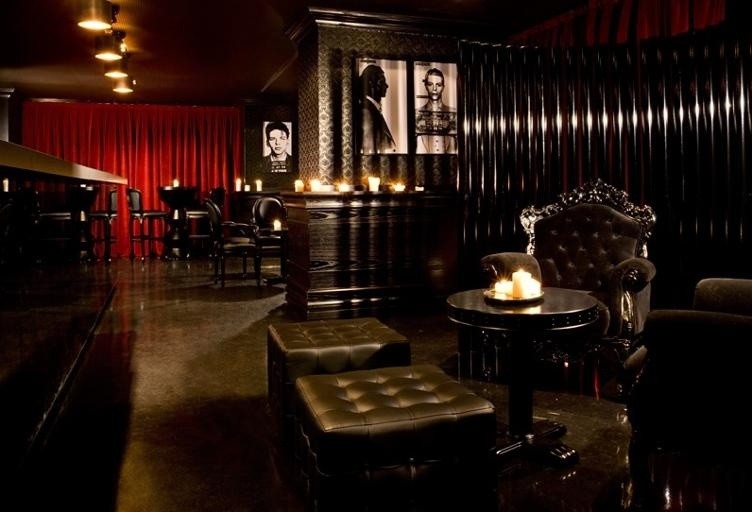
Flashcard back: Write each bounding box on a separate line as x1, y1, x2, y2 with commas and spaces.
244, 185, 250, 192
236, 177, 241, 192
257, 179, 262, 192
494, 272, 542, 298
174, 178, 179, 188
294, 176, 405, 192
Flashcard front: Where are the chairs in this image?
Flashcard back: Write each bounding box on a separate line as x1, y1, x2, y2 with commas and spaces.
617, 278, 752, 512
481, 177, 658, 404
12, 188, 289, 292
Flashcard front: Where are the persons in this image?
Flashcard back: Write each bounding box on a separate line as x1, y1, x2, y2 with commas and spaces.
415, 69, 458, 154
360, 64, 397, 153
263, 122, 294, 173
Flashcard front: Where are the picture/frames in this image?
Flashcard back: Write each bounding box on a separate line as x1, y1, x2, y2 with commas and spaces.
353, 53, 465, 157
260, 118, 295, 176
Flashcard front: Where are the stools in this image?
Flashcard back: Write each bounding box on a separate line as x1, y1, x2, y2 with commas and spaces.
266, 316, 411, 441
292, 362, 498, 512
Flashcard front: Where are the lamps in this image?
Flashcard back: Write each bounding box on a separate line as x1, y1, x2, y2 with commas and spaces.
76, 0, 134, 95
